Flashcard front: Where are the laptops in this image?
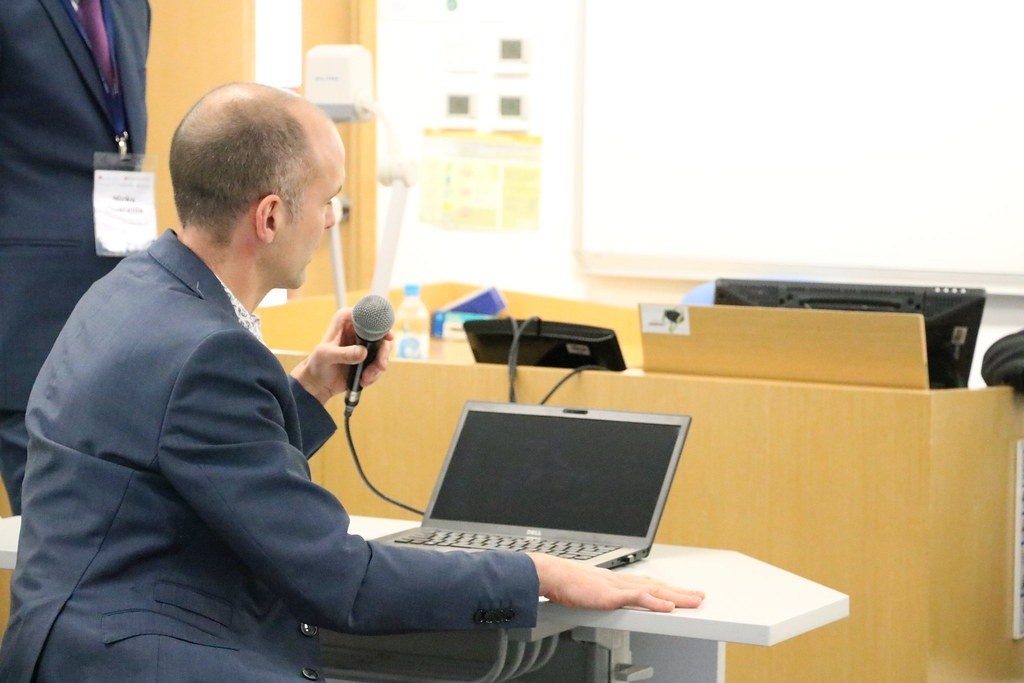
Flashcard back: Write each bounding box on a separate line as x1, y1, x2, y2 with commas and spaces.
362, 400, 691, 572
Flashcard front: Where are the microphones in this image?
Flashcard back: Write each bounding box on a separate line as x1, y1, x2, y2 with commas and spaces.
343, 295, 395, 414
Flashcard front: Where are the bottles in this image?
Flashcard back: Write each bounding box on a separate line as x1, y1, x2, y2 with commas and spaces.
391, 285, 430, 361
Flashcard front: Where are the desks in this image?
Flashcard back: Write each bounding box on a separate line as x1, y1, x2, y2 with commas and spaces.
0, 514, 853, 683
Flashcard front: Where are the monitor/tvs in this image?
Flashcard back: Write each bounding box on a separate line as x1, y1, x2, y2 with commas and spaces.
713, 276, 988, 394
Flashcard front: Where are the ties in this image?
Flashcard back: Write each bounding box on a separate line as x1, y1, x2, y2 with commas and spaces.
76, 0, 115, 88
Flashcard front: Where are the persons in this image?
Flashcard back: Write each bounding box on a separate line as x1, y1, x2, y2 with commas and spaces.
1, 79, 705, 683
0, 0, 152, 515
